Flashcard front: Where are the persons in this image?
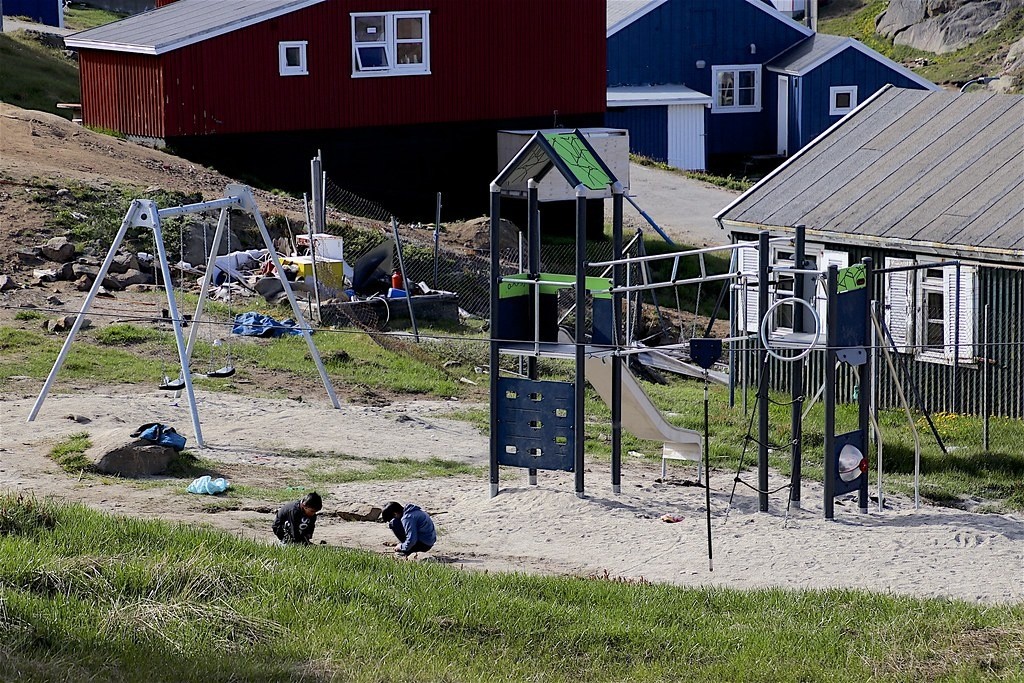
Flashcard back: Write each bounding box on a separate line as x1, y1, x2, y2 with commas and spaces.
272, 492, 322, 546
382, 502, 437, 559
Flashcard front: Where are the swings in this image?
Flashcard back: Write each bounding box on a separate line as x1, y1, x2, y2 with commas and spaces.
202, 211, 235, 378
152, 215, 186, 391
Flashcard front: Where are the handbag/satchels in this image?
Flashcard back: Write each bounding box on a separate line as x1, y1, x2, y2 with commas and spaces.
129, 423, 186, 451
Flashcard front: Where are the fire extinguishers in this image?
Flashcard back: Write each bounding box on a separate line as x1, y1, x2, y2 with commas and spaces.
391, 268, 402, 289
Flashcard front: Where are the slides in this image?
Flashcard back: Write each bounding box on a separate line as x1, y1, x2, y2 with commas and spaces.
557, 327, 702, 462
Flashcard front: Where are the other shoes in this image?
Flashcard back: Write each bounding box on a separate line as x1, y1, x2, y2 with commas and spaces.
301, 540, 316, 546
393, 552, 408, 559
